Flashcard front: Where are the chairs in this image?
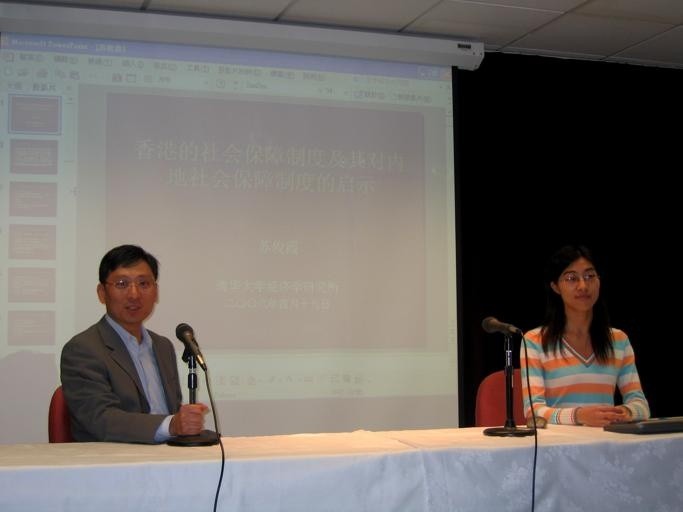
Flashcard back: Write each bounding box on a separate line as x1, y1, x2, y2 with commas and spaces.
475, 369, 527, 427
48, 386, 73, 442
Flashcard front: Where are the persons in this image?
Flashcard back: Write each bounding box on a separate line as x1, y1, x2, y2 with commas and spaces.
519, 251, 650, 427
59, 246, 211, 445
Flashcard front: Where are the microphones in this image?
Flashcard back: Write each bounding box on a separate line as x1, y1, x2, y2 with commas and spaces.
176, 322, 207, 371
482, 317, 523, 334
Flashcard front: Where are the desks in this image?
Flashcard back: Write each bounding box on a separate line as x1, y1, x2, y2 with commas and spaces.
0, 420, 682, 512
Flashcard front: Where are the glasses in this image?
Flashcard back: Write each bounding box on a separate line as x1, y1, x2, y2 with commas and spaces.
107, 281, 151, 289
560, 274, 599, 283
